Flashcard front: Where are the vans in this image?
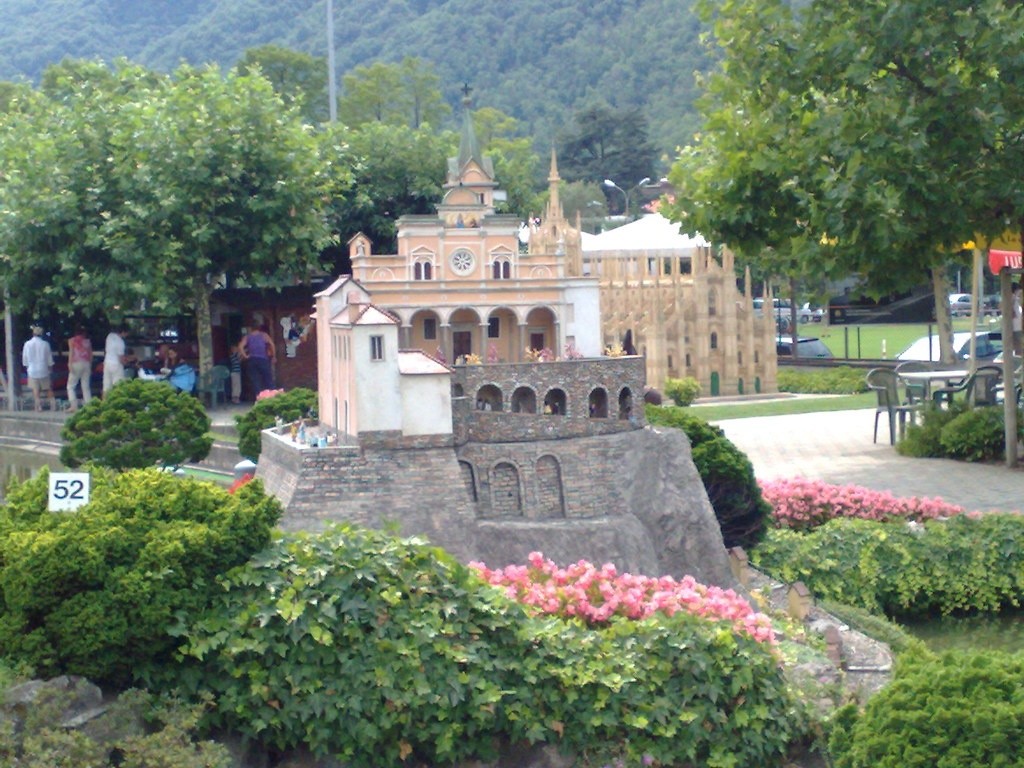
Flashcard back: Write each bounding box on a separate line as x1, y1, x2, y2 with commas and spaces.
776, 336, 834, 358
754, 298, 800, 320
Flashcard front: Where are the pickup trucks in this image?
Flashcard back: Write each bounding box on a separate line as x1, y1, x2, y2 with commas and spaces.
897, 332, 1003, 361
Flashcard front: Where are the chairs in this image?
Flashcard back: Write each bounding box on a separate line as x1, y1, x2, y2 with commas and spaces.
196, 365, 230, 411
865, 360, 1024, 447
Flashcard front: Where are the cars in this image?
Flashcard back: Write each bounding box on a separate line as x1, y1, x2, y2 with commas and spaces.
934, 294, 973, 319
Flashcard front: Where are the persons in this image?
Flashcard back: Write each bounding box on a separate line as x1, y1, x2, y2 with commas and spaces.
63, 323, 92, 413
22, 326, 57, 411
229, 319, 277, 404
101, 323, 131, 400
163, 347, 187, 369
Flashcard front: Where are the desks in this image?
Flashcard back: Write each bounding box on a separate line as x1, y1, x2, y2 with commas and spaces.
901, 371, 970, 411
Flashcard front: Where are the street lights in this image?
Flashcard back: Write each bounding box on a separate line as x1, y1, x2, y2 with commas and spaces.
603, 178, 651, 224
